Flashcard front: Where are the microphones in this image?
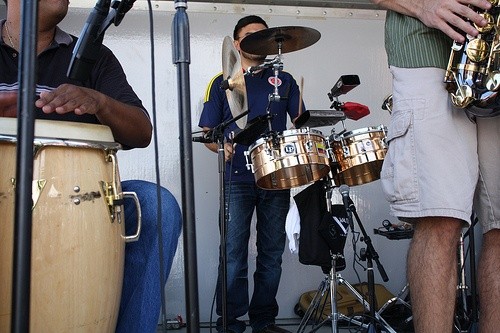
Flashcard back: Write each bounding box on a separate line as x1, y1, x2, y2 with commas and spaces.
67, 0, 111, 82
339, 185, 350, 217
191, 136, 227, 143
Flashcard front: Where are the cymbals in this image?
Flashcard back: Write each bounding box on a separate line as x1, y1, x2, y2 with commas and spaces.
240, 26, 321, 55
222, 36, 249, 129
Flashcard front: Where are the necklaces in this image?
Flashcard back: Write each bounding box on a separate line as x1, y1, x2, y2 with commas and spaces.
5, 21, 16, 49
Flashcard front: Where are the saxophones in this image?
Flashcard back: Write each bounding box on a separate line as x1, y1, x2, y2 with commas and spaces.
443, 0, 500, 117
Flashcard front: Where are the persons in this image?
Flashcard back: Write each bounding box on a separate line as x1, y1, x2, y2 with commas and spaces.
374, 0, 500, 333
198, 15, 307, 333
0, 0, 183, 333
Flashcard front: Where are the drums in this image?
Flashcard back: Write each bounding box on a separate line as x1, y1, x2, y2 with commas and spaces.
327, 124, 389, 186
0, 115, 125, 333
248, 128, 330, 190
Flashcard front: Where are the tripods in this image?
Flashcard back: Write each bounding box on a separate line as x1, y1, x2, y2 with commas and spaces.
293, 183, 477, 333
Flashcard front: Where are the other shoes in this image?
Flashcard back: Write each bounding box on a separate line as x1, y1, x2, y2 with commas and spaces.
253, 326, 293, 333
